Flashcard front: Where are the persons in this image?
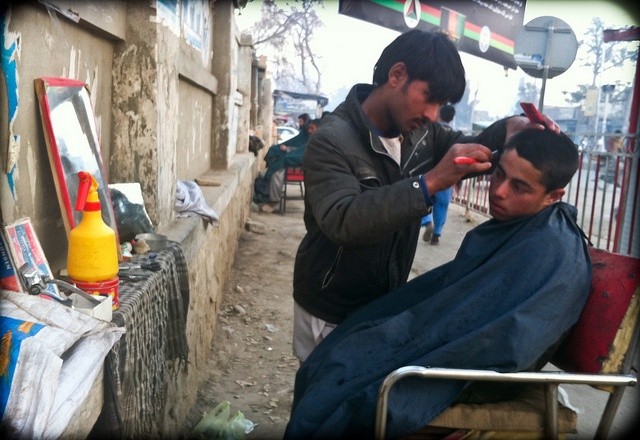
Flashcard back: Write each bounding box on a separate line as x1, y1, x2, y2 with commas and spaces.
285, 129, 592, 440
59, 156, 110, 229
264, 114, 310, 169
293, 30, 560, 378
607, 130, 624, 152
421, 105, 455, 245
262, 120, 322, 213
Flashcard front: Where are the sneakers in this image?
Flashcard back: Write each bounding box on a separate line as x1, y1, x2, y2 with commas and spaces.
424, 225, 433, 241
431, 235, 438, 245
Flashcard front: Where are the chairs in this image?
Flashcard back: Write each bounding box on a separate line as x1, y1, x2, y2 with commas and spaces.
280, 165, 307, 213
375, 245, 640, 439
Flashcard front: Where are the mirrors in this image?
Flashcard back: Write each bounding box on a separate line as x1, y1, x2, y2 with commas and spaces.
34, 76, 122, 262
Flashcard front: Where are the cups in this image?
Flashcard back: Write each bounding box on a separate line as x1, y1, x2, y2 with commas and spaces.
135, 233, 168, 252
